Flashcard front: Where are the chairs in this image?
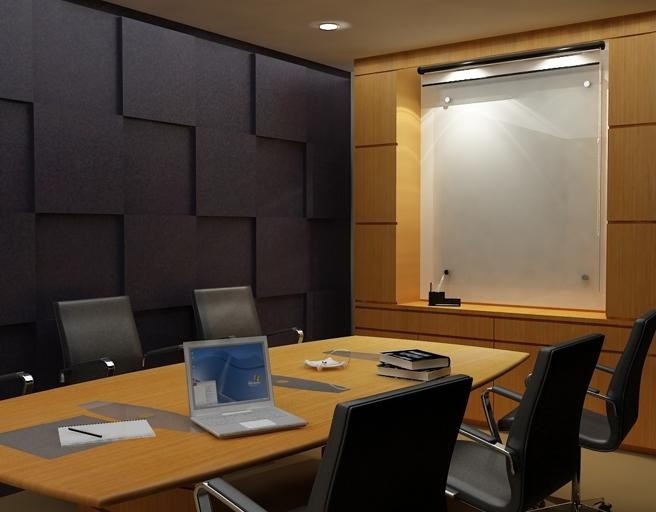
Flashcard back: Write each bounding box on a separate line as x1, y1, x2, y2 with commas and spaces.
0, 310, 530, 512
482, 292, 655, 510
444, 329, 605, 510
44, 294, 185, 389
0, 371, 35, 396
183, 285, 303, 348
192, 373, 472, 510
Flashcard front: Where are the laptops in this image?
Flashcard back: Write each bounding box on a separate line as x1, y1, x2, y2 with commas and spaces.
183, 335, 309, 439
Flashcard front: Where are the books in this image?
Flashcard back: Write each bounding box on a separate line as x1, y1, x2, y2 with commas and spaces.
57, 419, 156, 448
378, 346, 450, 370
377, 362, 451, 381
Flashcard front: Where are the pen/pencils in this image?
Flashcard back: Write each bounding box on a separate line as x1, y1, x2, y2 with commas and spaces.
68, 428, 103, 437
